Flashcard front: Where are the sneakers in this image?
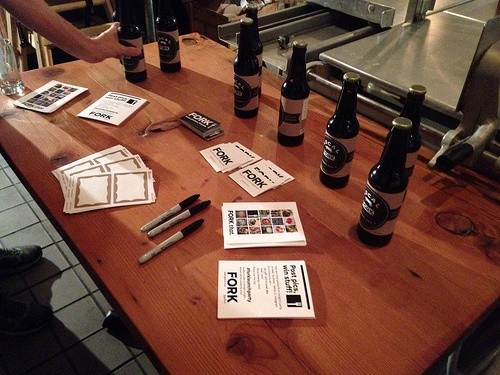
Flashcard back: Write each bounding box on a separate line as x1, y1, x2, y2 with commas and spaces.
0, 299, 54, 334
0, 245, 43, 275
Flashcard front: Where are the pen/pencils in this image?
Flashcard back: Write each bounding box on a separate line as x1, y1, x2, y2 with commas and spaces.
147, 199, 212, 237
139, 218, 205, 264
140, 193, 201, 232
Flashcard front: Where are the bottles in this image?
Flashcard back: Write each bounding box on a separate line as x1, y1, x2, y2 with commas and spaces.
357, 116, 413, 248
117, 0, 147, 84
276, 39, 311, 148
318, 70, 362, 191
155, 0, 182, 73
233, 17, 262, 118
245, 6, 263, 98
399, 84, 427, 177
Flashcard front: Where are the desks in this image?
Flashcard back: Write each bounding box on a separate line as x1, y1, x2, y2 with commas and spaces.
0, 31, 500, 374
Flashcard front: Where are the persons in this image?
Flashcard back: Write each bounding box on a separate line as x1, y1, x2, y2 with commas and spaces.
0, 0, 142, 336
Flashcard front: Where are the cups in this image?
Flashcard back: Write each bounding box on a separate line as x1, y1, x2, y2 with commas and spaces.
0, 37, 25, 96
